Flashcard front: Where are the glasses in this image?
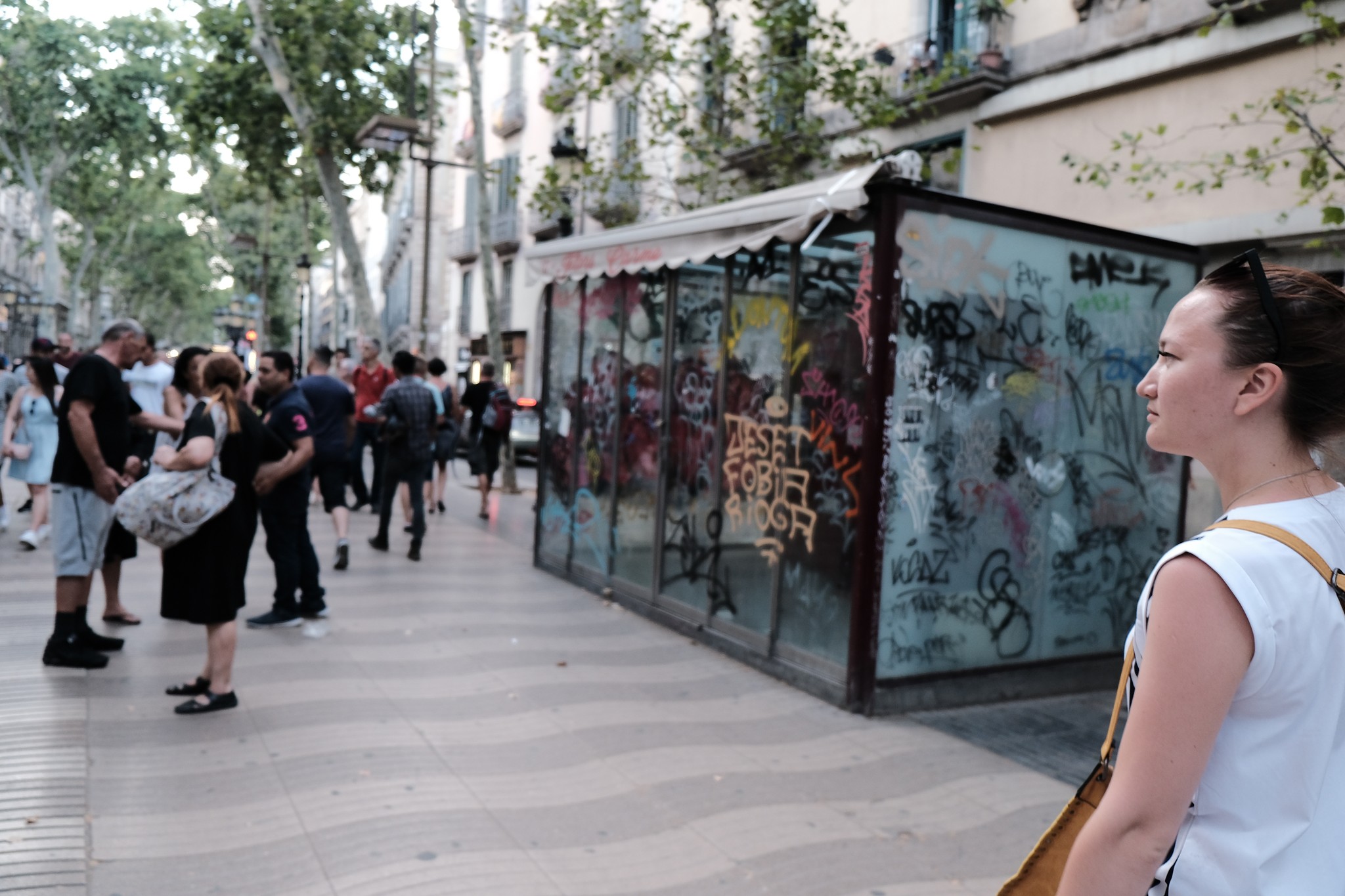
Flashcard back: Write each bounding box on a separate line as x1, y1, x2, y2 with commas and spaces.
1204, 248, 1287, 362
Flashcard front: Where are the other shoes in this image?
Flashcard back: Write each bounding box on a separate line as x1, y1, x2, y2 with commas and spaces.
174, 688, 238, 714
368, 535, 387, 550
19, 529, 37, 550
166, 676, 211, 696
333, 542, 348, 570
42, 633, 109, 668
248, 595, 326, 629
350, 493, 370, 512
74, 625, 124, 651
479, 513, 488, 519
404, 525, 413, 532
437, 500, 445, 512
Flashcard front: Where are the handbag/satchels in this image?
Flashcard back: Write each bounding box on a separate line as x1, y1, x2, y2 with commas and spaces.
996, 738, 1116, 896
434, 417, 461, 460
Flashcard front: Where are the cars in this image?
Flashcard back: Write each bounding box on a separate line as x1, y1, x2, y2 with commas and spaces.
508, 405, 540, 466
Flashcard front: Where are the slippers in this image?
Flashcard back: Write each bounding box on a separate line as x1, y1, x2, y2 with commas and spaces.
103, 612, 140, 624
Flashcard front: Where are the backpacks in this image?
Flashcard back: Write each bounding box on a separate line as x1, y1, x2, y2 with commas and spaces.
474, 384, 514, 461
114, 397, 237, 550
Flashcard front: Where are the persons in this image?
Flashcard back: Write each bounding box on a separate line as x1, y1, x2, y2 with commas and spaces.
904, 38, 939, 90
42, 318, 186, 669
1059, 247, 1345, 896
369, 348, 437, 562
247, 350, 327, 628
459, 363, 511, 518
151, 352, 268, 715
0, 335, 466, 626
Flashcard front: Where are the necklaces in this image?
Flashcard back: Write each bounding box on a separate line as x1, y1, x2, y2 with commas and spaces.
1223, 466, 1320, 513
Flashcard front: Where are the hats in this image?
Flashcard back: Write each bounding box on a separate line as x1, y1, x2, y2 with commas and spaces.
32, 338, 62, 351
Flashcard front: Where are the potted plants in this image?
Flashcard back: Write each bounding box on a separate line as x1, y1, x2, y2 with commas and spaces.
977, 0, 1015, 68
